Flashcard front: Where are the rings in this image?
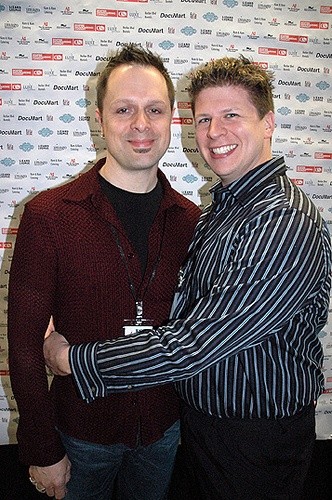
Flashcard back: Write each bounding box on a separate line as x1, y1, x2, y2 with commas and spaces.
29, 477, 36, 485
35, 483, 46, 493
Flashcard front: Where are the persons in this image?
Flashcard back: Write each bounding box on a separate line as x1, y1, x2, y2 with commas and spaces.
7, 44, 203, 500
42, 59, 332, 500
